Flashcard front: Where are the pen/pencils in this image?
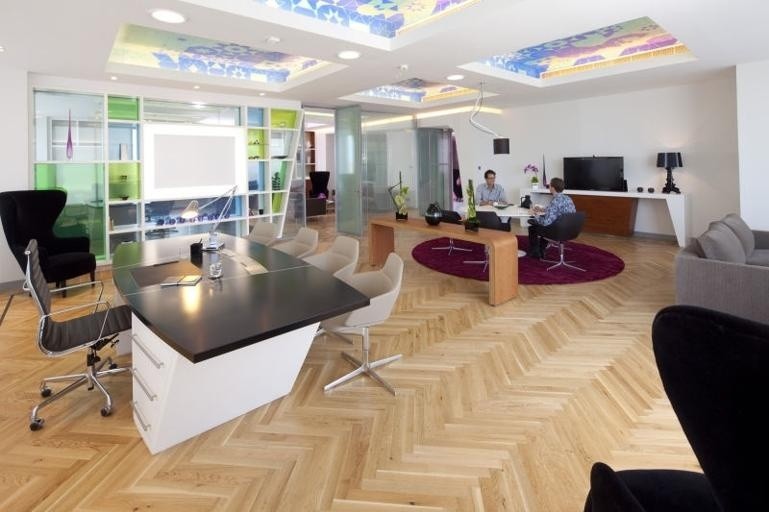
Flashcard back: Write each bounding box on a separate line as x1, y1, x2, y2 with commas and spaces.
153, 261, 180, 267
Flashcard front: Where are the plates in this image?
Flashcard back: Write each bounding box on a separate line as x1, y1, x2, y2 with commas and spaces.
494, 205, 509, 211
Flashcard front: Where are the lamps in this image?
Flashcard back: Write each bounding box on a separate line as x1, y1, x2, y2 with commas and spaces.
469, 80, 511, 155
656, 151, 684, 194
148, 6, 187, 25
339, 50, 360, 61
181, 185, 240, 251
446, 74, 464, 82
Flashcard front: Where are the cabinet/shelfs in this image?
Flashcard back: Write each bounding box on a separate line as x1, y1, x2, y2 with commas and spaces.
304, 130, 317, 197
32, 87, 306, 270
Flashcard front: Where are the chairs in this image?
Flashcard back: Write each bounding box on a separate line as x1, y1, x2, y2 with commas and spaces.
308, 171, 330, 197
583, 302, 769, 512
462, 211, 503, 273
321, 250, 404, 396
431, 210, 472, 256
20, 238, 133, 434
302, 234, 360, 345
0, 189, 98, 298
242, 221, 282, 245
269, 226, 319, 259
538, 212, 587, 272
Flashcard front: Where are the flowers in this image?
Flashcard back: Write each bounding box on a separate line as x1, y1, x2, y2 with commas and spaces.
523, 164, 540, 183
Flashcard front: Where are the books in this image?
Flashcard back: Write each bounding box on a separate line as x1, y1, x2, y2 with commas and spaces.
159, 275, 200, 286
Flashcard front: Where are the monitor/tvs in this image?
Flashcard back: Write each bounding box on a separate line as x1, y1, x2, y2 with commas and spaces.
563, 156, 624, 192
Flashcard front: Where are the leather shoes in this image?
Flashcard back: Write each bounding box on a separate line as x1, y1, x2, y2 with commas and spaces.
540, 252, 544, 258
528, 251, 541, 258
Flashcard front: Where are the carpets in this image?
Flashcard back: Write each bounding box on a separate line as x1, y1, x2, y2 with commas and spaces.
411, 236, 625, 285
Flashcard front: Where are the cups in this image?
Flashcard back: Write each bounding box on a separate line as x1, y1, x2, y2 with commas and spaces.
638, 188, 644, 192
493, 202, 499, 206
649, 188, 655, 193
207, 252, 224, 280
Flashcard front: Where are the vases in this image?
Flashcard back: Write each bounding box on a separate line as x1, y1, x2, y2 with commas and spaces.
532, 183, 538, 191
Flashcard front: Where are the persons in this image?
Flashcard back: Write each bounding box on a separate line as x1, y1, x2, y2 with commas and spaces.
526, 178, 575, 258
476, 170, 507, 225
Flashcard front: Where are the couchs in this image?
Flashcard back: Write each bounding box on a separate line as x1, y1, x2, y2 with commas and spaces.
673, 212, 768, 325
306, 198, 326, 216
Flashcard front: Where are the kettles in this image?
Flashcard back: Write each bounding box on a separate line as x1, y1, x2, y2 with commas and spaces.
521, 195, 533, 209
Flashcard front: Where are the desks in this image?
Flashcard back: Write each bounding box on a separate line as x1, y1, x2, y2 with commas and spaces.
368, 214, 519, 306
458, 205, 546, 258
532, 188, 686, 248
110, 232, 372, 459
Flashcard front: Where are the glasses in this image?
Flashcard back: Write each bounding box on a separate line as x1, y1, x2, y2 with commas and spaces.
487, 177, 495, 180
